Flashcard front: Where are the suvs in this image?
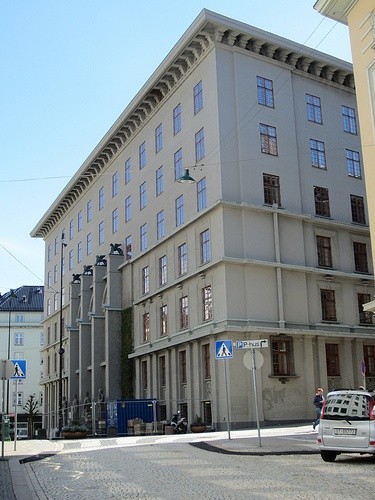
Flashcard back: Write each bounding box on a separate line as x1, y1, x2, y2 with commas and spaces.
316, 387, 375, 463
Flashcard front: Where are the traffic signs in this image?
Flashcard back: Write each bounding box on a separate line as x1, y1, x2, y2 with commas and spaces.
236, 339, 268, 348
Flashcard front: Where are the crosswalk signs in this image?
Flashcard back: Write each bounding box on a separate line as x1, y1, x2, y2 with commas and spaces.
9, 359, 27, 380
214, 340, 234, 359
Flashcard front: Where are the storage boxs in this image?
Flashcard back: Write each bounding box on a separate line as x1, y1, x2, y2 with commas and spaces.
106, 399, 158, 437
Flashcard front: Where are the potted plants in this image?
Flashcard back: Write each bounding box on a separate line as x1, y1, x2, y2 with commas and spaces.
59, 419, 89, 439
189, 414, 207, 432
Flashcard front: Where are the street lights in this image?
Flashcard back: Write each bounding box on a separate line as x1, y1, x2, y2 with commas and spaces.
0, 291, 18, 442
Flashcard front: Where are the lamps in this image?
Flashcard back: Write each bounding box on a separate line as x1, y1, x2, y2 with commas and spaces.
176, 167, 196, 184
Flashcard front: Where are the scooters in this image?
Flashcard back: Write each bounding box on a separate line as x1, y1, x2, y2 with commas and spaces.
172, 411, 188, 433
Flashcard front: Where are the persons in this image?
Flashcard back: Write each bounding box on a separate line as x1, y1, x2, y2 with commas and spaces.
62, 397, 68, 414
72, 394, 78, 414
312, 388, 325, 430
98, 389, 104, 409
83, 392, 91, 409
